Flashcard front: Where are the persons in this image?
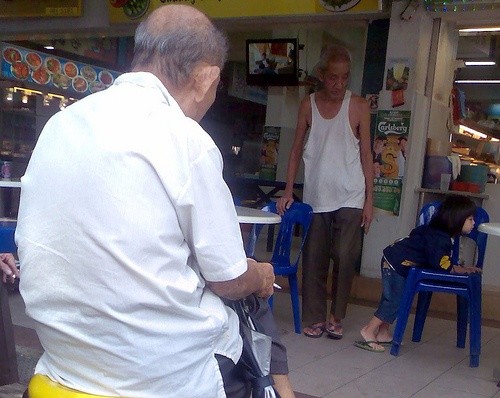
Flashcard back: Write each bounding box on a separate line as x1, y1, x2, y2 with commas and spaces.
276, 67, 373, 339
14, 3, 303, 398
249, 42, 295, 75
1, 252, 22, 388
359, 194, 481, 351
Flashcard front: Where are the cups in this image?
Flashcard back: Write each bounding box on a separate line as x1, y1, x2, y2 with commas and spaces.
440, 173, 451, 192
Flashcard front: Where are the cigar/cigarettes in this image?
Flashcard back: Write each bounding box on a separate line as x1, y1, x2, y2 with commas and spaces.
12, 269, 20, 278
273, 283, 283, 290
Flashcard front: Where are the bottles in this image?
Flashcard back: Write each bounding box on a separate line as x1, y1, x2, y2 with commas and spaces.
0, 143, 14, 181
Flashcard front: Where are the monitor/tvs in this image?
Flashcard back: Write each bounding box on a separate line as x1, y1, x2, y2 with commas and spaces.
246, 37, 299, 86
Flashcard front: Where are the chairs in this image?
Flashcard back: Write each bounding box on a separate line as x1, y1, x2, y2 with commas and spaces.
390, 200, 489, 368
246, 202, 313, 334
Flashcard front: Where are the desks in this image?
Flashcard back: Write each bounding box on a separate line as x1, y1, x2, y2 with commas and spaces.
415, 188, 489, 267
234, 205, 281, 224
234, 177, 304, 252
478, 223, 500, 236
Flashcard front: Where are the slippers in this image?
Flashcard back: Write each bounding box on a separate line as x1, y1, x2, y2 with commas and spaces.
325, 321, 343, 338
303, 326, 325, 338
381, 339, 393, 346
354, 339, 385, 352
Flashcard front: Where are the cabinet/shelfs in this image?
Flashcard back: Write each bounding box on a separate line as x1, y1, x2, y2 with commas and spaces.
0, 176, 21, 228
